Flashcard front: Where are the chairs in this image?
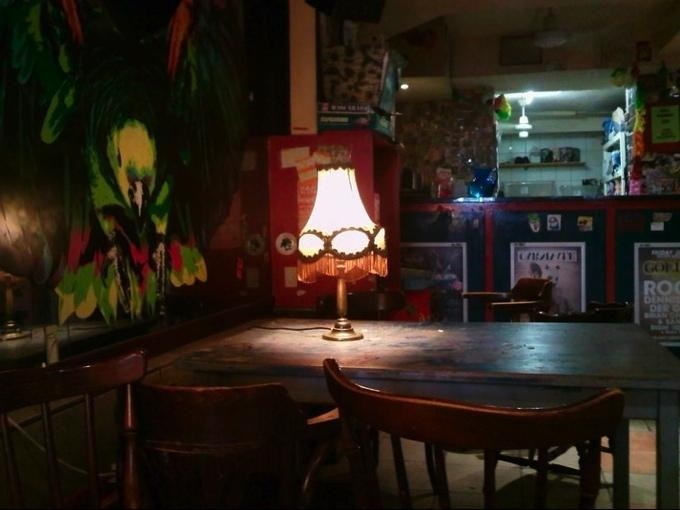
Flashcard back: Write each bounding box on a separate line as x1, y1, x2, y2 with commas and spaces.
121, 349, 385, 510
458, 276, 633, 321
0, 348, 147, 510
323, 358, 628, 510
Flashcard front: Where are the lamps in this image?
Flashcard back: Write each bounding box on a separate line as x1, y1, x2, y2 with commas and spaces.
298, 162, 388, 339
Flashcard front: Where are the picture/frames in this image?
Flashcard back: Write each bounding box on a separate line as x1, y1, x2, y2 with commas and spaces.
633, 241, 680, 350
509, 240, 587, 314
399, 239, 471, 320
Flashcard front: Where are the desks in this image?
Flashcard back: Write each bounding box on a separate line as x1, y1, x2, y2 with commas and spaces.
177, 320, 679, 510
